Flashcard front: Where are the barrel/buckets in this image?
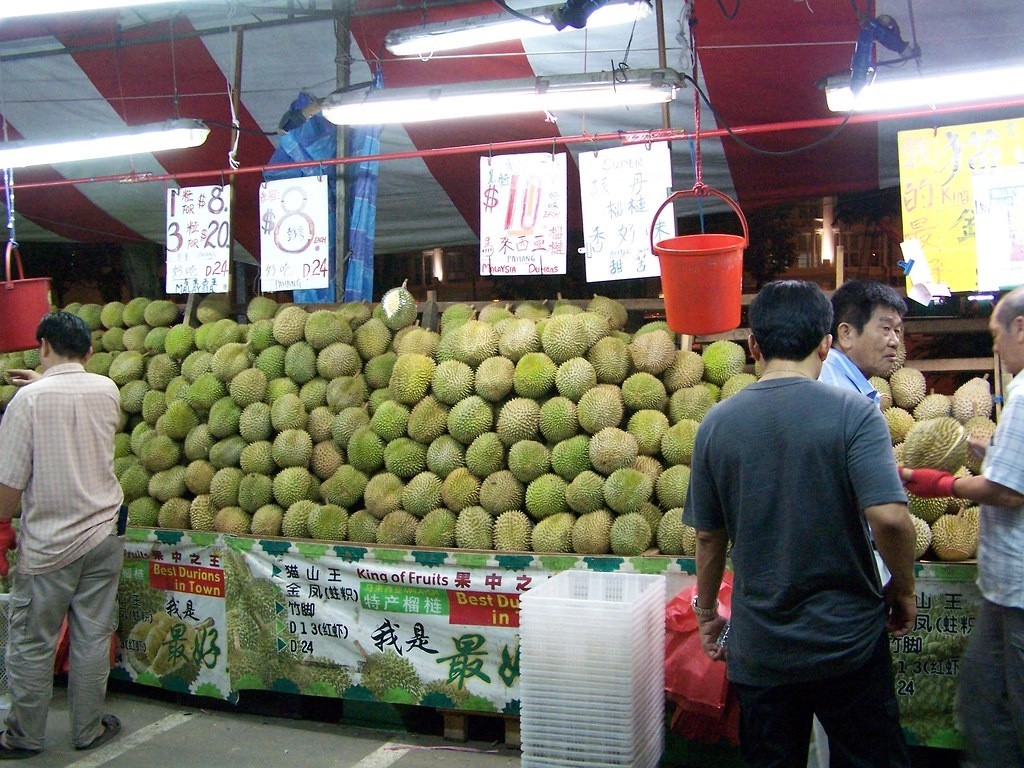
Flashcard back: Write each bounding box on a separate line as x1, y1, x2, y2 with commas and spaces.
650, 187, 749, 337
0, 242, 54, 353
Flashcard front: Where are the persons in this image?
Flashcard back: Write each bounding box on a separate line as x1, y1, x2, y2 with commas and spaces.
897, 286, 1024, 768
680, 278, 917, 768
811, 278, 899, 768
0, 311, 124, 761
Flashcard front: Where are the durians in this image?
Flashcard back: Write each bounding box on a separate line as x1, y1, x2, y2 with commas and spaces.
0, 286, 999, 561
101, 548, 470, 710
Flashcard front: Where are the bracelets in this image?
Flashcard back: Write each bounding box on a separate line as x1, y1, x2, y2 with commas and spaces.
692, 594, 720, 616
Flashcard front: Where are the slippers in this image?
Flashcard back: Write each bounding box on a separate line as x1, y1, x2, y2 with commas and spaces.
0, 730, 38, 760
85, 713, 121, 749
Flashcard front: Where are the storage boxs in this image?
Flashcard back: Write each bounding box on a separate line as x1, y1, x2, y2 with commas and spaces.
517, 570, 667, 768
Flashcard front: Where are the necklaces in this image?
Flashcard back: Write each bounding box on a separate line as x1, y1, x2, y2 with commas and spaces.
758, 370, 809, 379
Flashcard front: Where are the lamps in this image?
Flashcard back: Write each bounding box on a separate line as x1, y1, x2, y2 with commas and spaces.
383, 0, 653, 57
321, 67, 687, 126
824, 54, 1024, 112
0, 19, 210, 169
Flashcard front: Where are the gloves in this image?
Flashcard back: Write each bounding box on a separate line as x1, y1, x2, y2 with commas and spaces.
0, 520, 17, 577
